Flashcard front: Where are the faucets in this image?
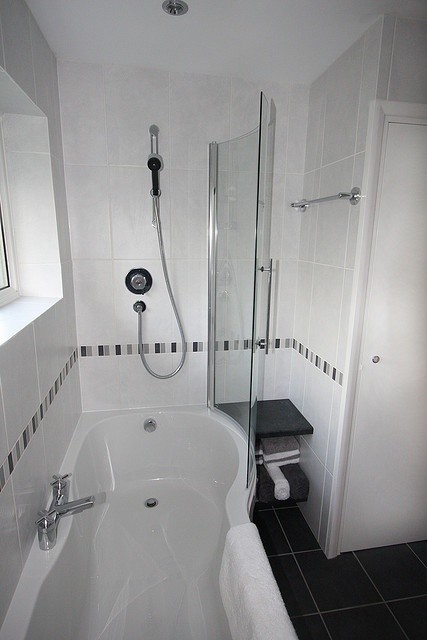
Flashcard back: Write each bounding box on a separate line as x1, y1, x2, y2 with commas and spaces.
54, 495, 97, 518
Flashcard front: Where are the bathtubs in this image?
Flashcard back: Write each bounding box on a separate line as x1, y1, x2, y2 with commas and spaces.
1, 405, 295, 635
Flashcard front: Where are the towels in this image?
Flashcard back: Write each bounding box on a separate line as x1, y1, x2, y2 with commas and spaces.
266, 463, 293, 502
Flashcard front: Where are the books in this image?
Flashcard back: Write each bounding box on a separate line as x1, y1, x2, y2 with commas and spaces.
255, 436, 301, 468
